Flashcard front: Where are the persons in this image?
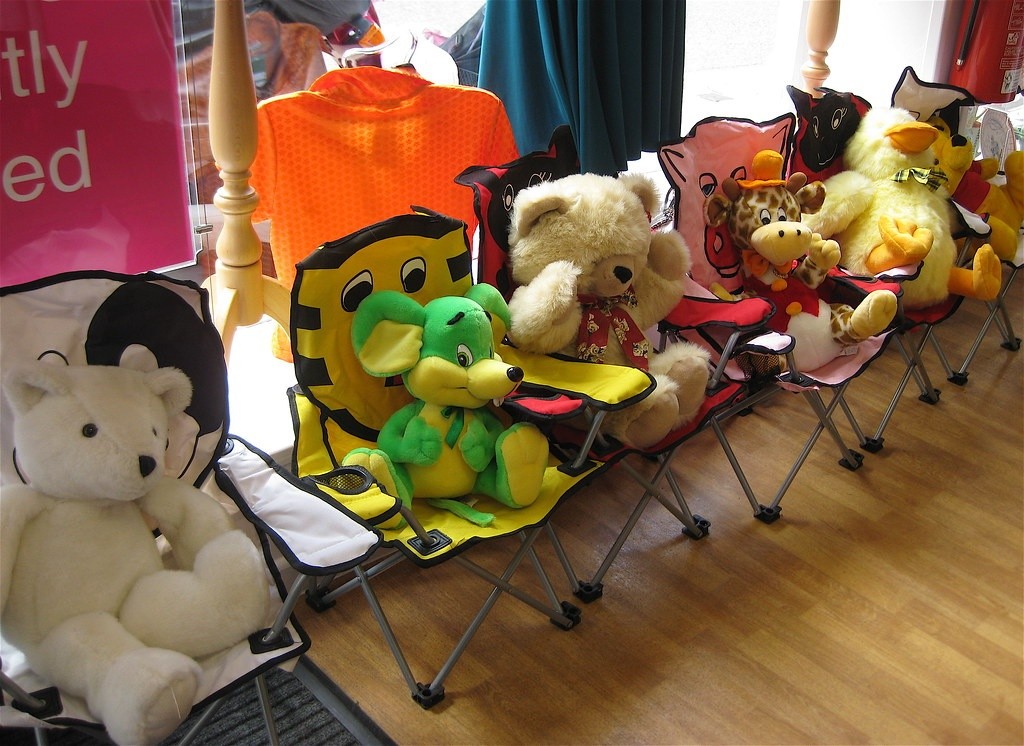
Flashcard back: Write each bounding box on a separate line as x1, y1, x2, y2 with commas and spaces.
180, 1, 373, 62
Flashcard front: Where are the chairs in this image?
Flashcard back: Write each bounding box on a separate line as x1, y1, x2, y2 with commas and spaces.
452, 124, 779, 604
785, 82, 991, 448
891, 66, 1023, 386
285, 204, 657, 709
657, 112, 925, 523
1, 268, 383, 746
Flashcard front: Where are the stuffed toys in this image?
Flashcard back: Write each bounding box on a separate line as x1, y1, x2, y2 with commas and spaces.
922, 114, 1024, 263
336, 283, 550, 529
0, 358, 268, 745
801, 105, 1002, 312
505, 170, 711, 452
704, 150, 898, 378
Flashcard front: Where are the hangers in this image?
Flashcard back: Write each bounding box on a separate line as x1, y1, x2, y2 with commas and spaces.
258, 32, 505, 117
